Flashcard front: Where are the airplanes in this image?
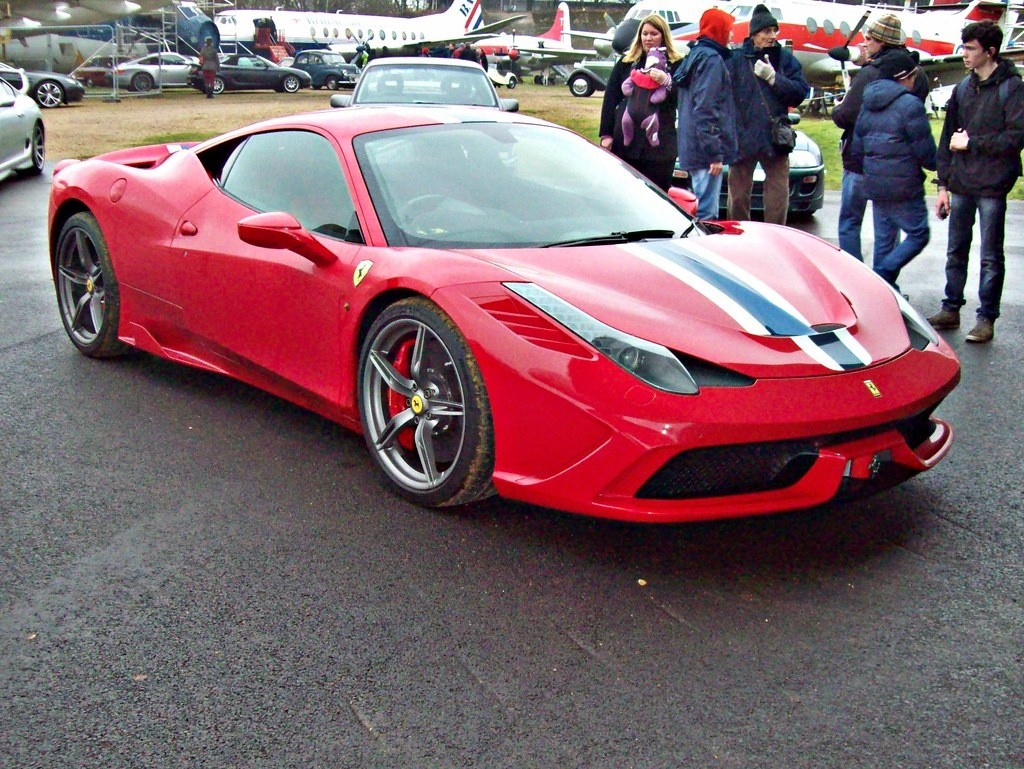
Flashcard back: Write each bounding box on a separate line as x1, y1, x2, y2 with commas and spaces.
472, 2, 576, 69
209, 0, 529, 54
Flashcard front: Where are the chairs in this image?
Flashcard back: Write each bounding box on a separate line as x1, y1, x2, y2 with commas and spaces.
377, 74, 404, 99
438, 77, 470, 105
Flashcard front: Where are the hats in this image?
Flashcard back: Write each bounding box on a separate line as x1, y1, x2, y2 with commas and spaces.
881, 50, 918, 81
868, 14, 901, 45
749, 4, 779, 37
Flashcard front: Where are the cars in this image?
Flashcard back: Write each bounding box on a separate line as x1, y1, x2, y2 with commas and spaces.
0, 76, 47, 184
673, 110, 826, 222
278, 57, 295, 68
288, 50, 361, 91
0, 61, 86, 108
73, 55, 131, 89
329, 57, 519, 114
564, 59, 615, 97
924, 83, 958, 119
107, 51, 201, 91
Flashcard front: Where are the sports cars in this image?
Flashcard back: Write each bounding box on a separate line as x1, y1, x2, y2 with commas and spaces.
186, 54, 312, 95
47, 102, 966, 532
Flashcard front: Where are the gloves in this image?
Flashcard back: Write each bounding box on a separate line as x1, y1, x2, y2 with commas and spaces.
754, 54, 775, 84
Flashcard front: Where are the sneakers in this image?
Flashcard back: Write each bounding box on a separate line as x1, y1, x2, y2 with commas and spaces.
926, 309, 961, 328
967, 319, 994, 342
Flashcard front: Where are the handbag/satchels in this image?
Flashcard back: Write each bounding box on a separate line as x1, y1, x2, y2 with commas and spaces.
200, 56, 204, 65
770, 114, 798, 151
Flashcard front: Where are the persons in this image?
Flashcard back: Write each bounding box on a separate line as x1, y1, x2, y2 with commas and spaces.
200, 37, 220, 99
356, 44, 392, 70
728, 4, 810, 229
830, 16, 930, 265
673, 9, 739, 222
267, 16, 276, 45
599, 15, 685, 194
422, 42, 488, 78
935, 19, 1024, 341
849, 50, 937, 303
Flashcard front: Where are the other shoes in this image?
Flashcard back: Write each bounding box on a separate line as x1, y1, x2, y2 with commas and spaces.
207, 95, 214, 98
